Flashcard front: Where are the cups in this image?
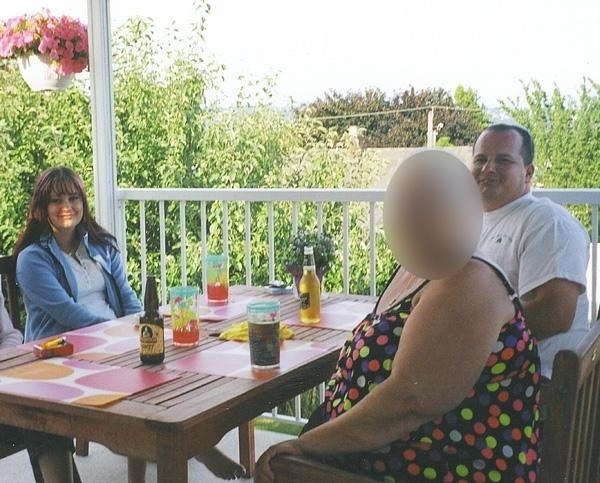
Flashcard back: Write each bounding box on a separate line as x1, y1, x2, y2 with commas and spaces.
170, 285, 200, 348
204, 255, 230, 304
244, 299, 282, 370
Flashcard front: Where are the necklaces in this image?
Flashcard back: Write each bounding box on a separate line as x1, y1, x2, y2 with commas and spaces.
389, 275, 423, 306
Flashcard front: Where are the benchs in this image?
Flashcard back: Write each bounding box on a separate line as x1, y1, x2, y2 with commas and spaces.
254, 307, 600, 483
1, 256, 89, 457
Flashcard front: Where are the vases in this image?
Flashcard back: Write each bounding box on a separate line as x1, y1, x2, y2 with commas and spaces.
14, 49, 75, 92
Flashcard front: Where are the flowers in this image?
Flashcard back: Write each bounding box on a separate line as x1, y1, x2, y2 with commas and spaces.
0, 7, 90, 76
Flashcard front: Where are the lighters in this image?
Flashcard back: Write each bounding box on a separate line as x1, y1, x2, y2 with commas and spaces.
40, 337, 66, 349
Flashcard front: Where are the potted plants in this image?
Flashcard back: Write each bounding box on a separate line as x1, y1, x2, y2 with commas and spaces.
281, 225, 338, 304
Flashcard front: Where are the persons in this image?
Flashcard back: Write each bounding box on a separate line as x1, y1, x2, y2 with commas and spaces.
254, 254, 544, 482
13, 164, 247, 482
1, 290, 82, 482
470, 124, 591, 383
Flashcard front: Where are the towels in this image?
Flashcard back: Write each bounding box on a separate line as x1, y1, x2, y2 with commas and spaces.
219, 319, 294, 342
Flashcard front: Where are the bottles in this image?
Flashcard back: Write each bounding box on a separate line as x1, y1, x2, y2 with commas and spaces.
299, 244, 323, 324
138, 275, 166, 364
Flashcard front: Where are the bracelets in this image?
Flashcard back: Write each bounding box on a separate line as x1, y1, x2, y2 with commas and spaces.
295, 441, 309, 458
